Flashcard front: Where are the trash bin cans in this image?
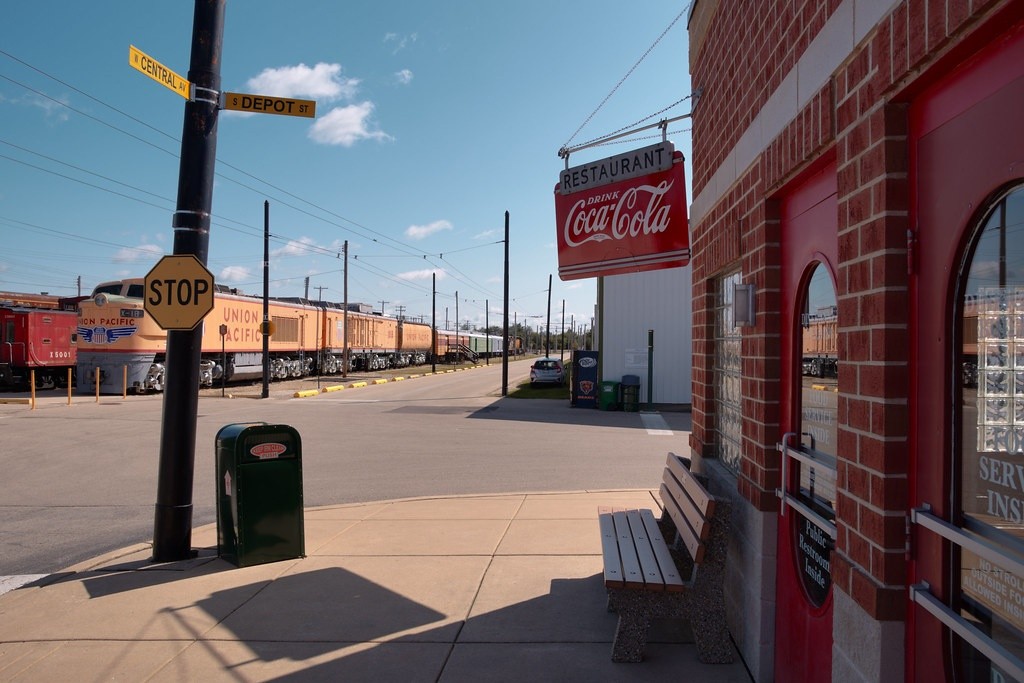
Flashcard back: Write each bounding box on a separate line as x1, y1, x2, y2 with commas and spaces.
621, 375, 642, 411
598, 380, 620, 411
215, 421, 306, 568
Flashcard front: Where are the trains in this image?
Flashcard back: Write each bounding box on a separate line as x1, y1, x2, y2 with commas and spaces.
74, 278, 502, 397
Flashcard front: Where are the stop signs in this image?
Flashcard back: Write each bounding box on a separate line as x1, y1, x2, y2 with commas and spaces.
142, 252, 217, 330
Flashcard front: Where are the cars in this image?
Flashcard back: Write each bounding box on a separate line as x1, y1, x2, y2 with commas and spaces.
529, 358, 565, 387
802, 357, 838, 378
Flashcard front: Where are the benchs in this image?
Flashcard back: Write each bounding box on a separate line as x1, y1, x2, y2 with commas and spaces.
596, 451, 734, 666
